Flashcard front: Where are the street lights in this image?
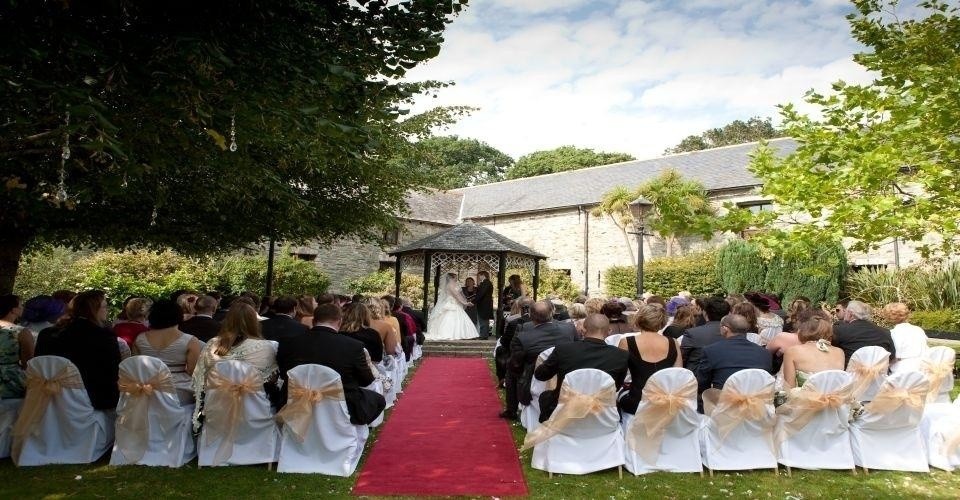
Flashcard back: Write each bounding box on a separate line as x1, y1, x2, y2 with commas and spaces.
626, 195, 655, 296
891, 184, 912, 272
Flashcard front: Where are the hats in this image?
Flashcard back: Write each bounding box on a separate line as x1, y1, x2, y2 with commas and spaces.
20, 294, 66, 323
743, 290, 783, 311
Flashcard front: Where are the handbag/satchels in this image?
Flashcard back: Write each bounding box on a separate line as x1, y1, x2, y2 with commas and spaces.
500, 310, 512, 321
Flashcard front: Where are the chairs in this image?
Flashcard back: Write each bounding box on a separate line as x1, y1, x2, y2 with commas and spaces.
518, 370, 627, 478
849, 370, 928, 476
198, 360, 282, 468
277, 366, 370, 477
494, 331, 955, 400
777, 369, 853, 475
10, 355, 115, 466
2, 330, 423, 427
622, 368, 701, 475
697, 368, 779, 474
106, 353, 204, 469
925, 394, 959, 474
0, 394, 28, 458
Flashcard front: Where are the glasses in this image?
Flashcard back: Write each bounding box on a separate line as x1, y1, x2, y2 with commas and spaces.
835, 309, 845, 312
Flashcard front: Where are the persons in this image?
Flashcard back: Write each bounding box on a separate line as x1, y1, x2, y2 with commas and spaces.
422, 273, 480, 341
469, 271, 494, 340
497, 291, 932, 427
1, 289, 425, 434
461, 278, 478, 326
496, 273, 524, 336
